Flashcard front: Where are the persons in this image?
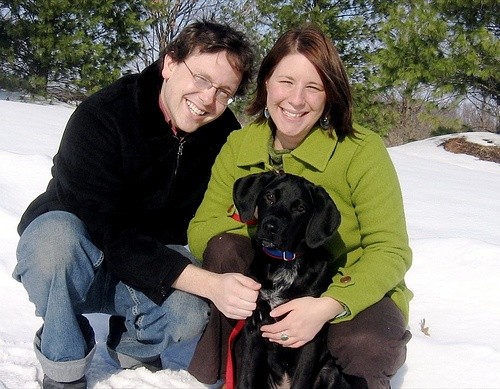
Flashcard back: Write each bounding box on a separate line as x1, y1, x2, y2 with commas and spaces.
12, 22, 261, 389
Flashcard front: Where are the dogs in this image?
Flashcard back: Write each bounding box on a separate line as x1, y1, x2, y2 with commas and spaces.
232, 169, 367, 389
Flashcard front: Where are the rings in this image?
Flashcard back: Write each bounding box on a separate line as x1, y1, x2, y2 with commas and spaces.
280, 333, 288, 340
186, 28, 414, 389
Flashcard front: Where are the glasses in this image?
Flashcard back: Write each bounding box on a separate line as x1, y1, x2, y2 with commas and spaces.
182, 60, 235, 106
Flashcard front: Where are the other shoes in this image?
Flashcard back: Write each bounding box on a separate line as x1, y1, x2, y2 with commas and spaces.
43, 372, 87, 389
128, 354, 163, 373
